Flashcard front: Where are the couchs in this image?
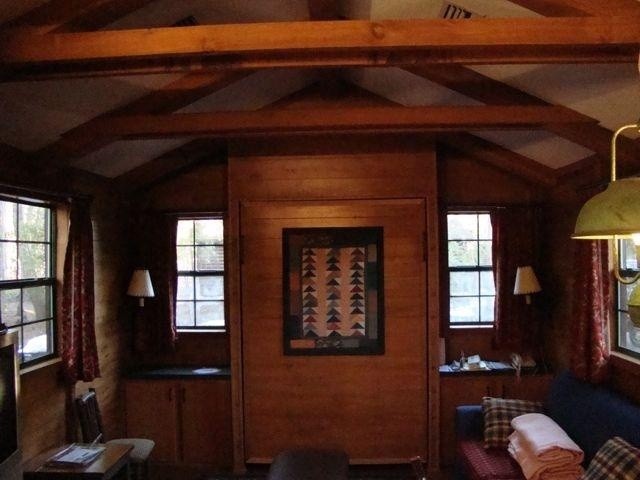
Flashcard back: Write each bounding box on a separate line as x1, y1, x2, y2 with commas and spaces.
453, 371, 639, 480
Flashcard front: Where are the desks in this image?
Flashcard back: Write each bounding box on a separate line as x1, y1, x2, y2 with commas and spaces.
24, 439, 135, 480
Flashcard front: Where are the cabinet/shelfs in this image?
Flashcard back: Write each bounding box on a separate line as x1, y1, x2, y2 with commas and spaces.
440, 362, 516, 409
225, 154, 443, 474
126, 367, 230, 470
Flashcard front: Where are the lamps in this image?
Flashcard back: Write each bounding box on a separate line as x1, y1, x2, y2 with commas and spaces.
570, 123, 640, 285
128, 269, 154, 308
513, 265, 542, 305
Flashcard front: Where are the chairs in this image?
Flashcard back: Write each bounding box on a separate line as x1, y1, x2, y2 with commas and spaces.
71, 387, 157, 480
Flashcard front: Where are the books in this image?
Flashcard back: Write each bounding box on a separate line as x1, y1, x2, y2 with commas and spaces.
45, 443, 106, 468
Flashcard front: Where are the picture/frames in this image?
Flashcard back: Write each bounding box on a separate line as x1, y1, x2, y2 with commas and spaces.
282, 227, 385, 356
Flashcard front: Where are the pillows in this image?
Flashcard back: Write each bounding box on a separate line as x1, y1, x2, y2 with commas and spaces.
480, 396, 539, 450
573, 434, 640, 480
